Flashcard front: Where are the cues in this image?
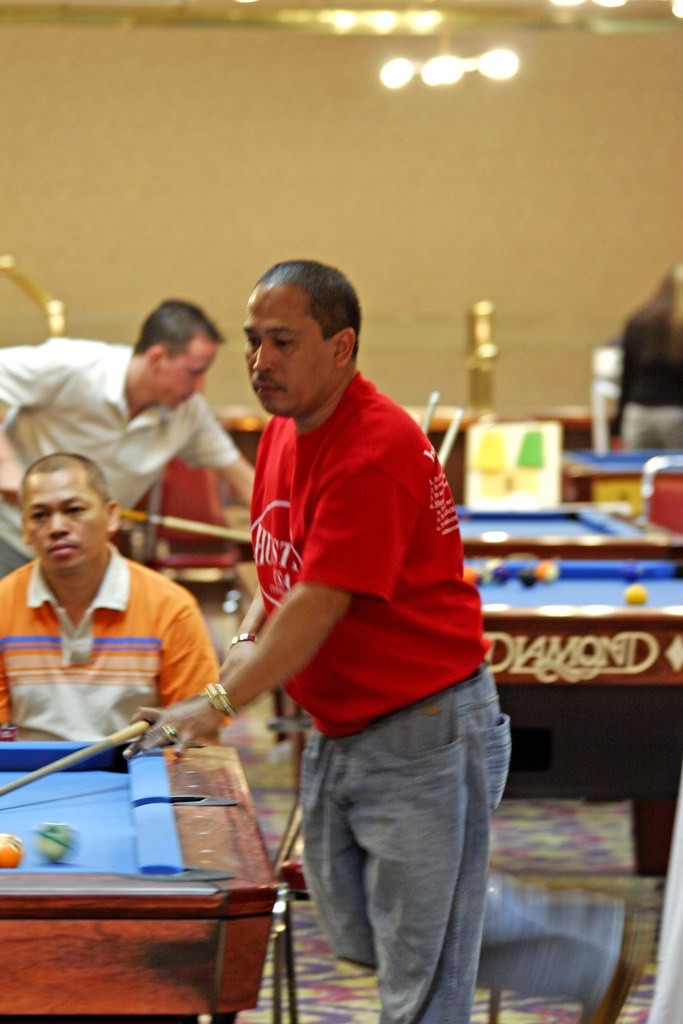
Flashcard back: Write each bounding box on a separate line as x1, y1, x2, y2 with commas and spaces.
119, 505, 253, 546
416, 391, 469, 471
0, 716, 156, 801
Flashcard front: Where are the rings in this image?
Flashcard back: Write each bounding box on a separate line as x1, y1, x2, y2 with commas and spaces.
161, 724, 182, 743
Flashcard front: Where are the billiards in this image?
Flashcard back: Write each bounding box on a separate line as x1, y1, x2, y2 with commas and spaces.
464, 558, 560, 587
625, 583, 646, 604
38, 825, 71, 861
1, 833, 25, 869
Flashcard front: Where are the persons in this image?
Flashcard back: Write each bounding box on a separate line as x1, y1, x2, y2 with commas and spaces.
0, 299, 256, 571
612, 263, 683, 437
126, 263, 642, 1024
0, 451, 228, 743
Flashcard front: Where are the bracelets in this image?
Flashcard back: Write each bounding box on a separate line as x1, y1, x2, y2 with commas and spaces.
228, 633, 258, 648
200, 683, 238, 719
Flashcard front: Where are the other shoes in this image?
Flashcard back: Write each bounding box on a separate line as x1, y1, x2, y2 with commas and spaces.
581, 954, 639, 1024
